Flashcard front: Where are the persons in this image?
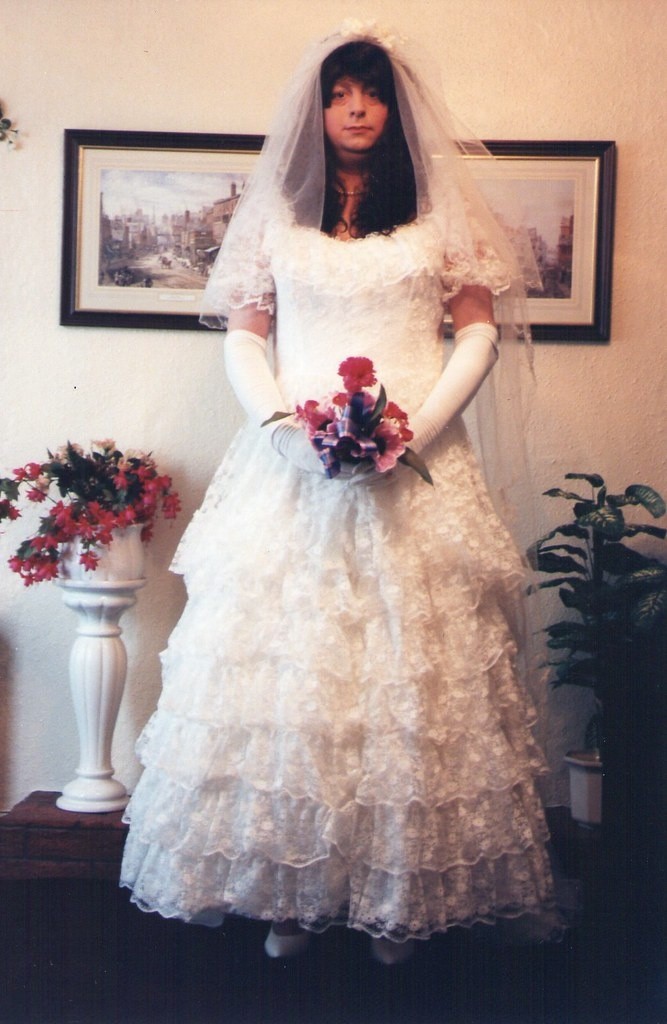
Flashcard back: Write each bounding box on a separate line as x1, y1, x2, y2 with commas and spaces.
116, 18, 555, 961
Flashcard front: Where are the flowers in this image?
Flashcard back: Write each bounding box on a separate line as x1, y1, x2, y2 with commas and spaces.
293, 357, 437, 490
0, 438, 183, 588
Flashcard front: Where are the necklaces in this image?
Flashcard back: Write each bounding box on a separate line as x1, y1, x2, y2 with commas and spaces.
331, 183, 370, 196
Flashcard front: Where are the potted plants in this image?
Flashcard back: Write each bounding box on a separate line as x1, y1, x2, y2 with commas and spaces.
517, 473, 667, 829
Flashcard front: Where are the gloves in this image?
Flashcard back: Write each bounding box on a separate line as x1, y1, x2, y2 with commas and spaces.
349, 323, 500, 493
223, 330, 355, 478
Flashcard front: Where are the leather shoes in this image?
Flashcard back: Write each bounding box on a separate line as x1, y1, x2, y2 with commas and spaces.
264, 922, 308, 958
371, 937, 416, 964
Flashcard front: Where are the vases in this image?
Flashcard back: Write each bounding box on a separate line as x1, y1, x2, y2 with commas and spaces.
56, 522, 147, 813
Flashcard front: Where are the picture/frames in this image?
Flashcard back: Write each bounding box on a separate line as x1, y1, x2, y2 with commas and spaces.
59, 129, 269, 333
432, 139, 616, 344
